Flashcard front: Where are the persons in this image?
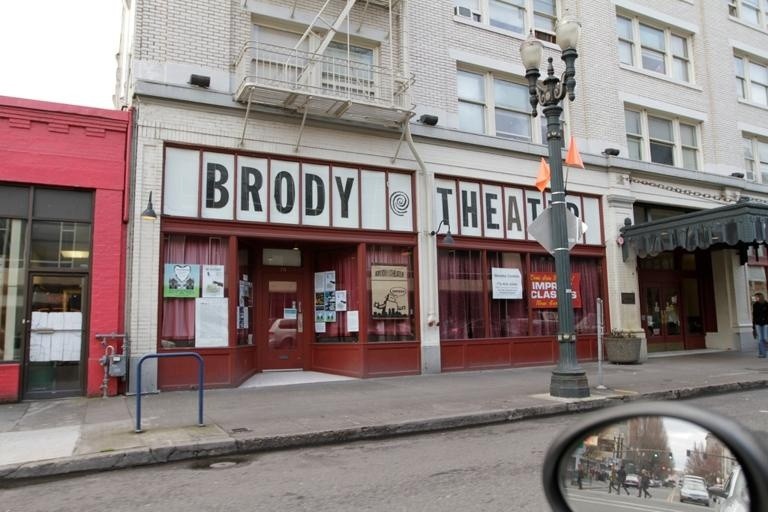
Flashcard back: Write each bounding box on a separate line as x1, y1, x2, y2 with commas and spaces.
751, 291, 767, 359
575, 460, 652, 501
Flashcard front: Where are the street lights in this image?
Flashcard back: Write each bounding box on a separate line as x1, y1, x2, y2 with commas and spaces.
519, 9, 590, 397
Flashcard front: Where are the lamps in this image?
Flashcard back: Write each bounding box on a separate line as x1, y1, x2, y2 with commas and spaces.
142, 192, 155, 221
433, 220, 453, 246
732, 172, 746, 178
602, 149, 619, 155
418, 114, 438, 125
189, 73, 211, 88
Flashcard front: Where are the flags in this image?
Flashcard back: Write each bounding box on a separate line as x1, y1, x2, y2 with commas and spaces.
564, 134, 584, 170
533, 156, 552, 193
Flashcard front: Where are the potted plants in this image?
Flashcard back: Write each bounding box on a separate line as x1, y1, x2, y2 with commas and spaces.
604, 330, 643, 364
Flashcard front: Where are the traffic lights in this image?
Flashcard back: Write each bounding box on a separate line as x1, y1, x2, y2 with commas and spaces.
654, 452, 658, 458
668, 453, 673, 460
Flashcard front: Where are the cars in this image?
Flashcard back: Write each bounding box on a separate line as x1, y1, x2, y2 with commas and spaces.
624, 475, 676, 489
678, 465, 751, 512
248, 319, 298, 344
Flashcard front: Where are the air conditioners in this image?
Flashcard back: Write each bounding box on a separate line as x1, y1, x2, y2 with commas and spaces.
455, 6, 474, 19
